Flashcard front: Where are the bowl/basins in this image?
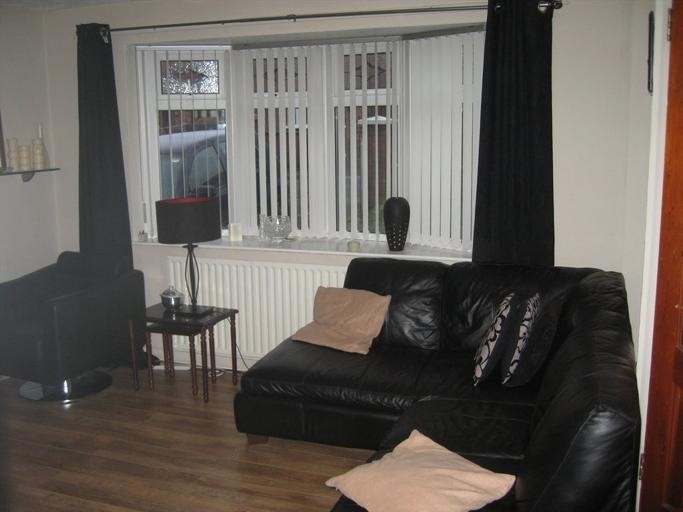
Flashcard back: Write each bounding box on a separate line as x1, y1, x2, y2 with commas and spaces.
162, 309, 181, 321
264, 216, 292, 239
159, 294, 185, 309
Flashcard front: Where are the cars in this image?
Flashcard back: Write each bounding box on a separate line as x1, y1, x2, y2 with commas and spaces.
156, 127, 280, 233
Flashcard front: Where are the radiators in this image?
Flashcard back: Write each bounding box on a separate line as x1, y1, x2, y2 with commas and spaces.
169, 256, 348, 363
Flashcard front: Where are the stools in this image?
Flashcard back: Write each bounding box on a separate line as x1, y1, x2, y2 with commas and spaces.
145, 323, 217, 397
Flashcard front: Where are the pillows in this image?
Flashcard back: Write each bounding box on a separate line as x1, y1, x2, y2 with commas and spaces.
472, 293, 523, 386
324, 428, 517, 511
498, 284, 567, 388
291, 286, 392, 356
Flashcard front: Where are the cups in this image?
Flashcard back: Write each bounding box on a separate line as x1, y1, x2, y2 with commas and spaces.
6, 136, 48, 173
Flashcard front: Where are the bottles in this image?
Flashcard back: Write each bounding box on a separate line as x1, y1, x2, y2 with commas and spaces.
257, 214, 268, 241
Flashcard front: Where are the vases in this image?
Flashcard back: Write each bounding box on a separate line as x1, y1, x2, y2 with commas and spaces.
382, 197, 410, 251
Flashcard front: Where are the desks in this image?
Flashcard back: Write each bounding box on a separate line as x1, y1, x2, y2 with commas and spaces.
119, 302, 239, 403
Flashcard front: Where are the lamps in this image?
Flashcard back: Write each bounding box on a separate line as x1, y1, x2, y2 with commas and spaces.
155, 197, 221, 318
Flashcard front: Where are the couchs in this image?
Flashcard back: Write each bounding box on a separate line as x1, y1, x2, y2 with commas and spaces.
233, 258, 641, 511
0, 251, 148, 400
